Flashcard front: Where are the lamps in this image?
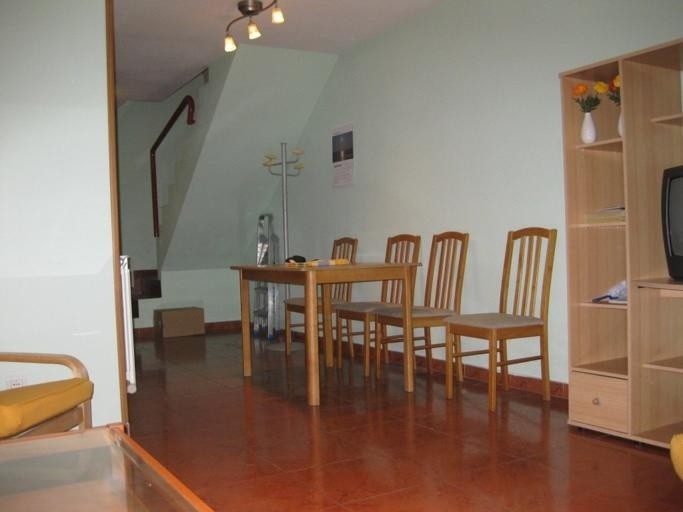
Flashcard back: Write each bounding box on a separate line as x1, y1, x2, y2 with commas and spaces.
221, 1, 286, 52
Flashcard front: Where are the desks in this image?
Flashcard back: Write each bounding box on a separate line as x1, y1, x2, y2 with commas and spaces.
228, 264, 422, 406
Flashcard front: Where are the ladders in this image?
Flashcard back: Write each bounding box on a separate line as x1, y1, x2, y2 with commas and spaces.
254, 213, 280, 341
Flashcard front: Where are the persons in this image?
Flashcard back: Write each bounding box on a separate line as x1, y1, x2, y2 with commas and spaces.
331, 136, 353, 163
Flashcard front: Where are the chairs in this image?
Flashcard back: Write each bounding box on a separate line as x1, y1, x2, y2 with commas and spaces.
443, 228, 557, 412
374, 231, 470, 393
332, 233, 422, 378
285, 237, 359, 357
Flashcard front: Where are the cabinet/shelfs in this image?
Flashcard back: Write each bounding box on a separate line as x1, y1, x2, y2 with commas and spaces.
557, 36, 681, 449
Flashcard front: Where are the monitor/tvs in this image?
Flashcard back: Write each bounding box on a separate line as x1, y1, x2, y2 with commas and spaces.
660, 164, 683, 285
